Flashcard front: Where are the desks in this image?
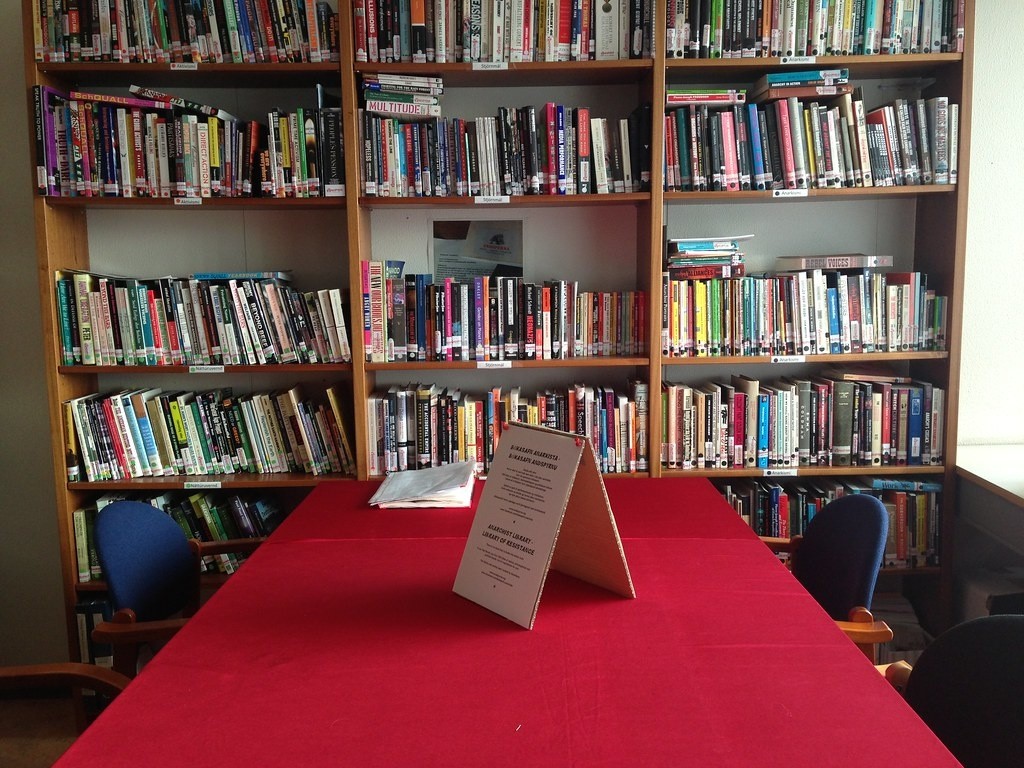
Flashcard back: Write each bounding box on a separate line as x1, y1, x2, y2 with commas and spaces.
50, 476, 966, 768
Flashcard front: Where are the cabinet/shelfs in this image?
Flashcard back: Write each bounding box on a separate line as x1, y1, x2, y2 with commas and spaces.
18, 0, 973, 668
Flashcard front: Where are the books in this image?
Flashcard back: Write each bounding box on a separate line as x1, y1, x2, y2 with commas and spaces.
361, 258, 645, 363
366, 459, 477, 510
719, 478, 944, 568
820, 365, 912, 383
54, 266, 353, 369
62, 381, 358, 483
366, 377, 649, 481
27, 1, 961, 60
73, 598, 120, 672
662, 230, 948, 358
30, 70, 959, 199
73, 492, 309, 584
660, 375, 947, 470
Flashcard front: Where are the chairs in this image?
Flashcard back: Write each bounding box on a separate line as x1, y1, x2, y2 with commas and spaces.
757, 494, 893, 665
91, 500, 264, 677
907, 614, 1024, 768
0, 659, 132, 768
